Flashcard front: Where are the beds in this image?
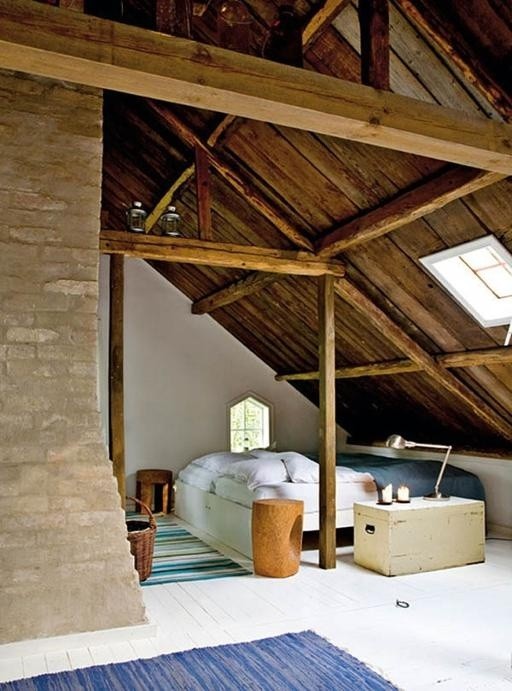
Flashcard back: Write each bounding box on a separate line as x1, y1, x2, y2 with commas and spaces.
173, 451, 488, 561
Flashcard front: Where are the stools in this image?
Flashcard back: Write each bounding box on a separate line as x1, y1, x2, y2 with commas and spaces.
251, 498, 304, 578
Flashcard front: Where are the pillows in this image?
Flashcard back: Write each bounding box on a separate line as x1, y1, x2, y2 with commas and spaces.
192, 450, 319, 491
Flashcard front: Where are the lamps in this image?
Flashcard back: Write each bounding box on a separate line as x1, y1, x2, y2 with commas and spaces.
386, 434, 452, 501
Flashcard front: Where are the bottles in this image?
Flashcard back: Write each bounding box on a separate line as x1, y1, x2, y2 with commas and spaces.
125, 201, 147, 235
160, 206, 182, 237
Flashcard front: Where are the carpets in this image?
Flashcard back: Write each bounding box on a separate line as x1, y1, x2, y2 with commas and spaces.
125, 509, 253, 586
0, 630, 401, 691
